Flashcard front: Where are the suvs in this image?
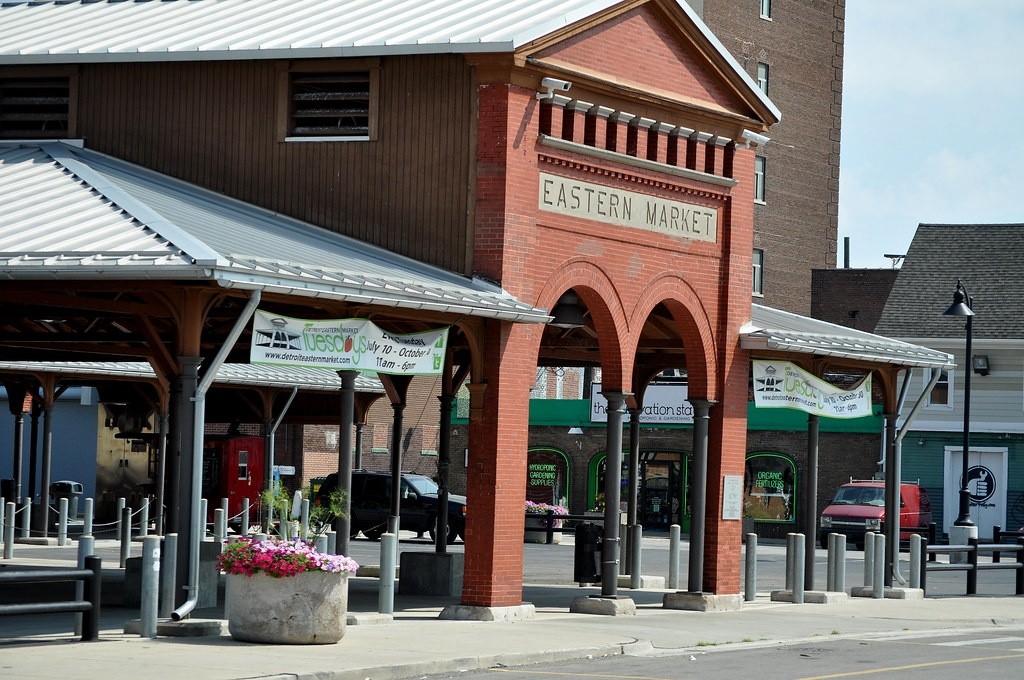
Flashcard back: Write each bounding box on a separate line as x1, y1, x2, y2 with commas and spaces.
314, 469, 467, 545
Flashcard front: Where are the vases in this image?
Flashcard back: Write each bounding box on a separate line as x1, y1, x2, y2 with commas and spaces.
225, 566, 349, 643
525, 516, 563, 543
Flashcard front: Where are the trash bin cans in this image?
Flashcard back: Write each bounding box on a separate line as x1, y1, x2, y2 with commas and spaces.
49, 480, 84, 522
575, 511, 628, 583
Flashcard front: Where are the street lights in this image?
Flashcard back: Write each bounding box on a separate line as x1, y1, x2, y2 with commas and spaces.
941, 278, 979, 565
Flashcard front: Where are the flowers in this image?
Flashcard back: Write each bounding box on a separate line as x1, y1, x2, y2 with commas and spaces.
216, 538, 360, 579
524, 499, 569, 528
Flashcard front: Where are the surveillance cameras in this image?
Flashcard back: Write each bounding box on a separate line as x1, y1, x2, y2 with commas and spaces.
541, 77, 572, 91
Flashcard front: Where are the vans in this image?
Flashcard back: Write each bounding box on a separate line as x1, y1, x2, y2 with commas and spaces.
818, 476, 932, 552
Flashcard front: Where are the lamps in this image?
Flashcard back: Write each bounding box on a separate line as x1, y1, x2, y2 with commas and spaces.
972, 355, 991, 376
568, 427, 584, 435
